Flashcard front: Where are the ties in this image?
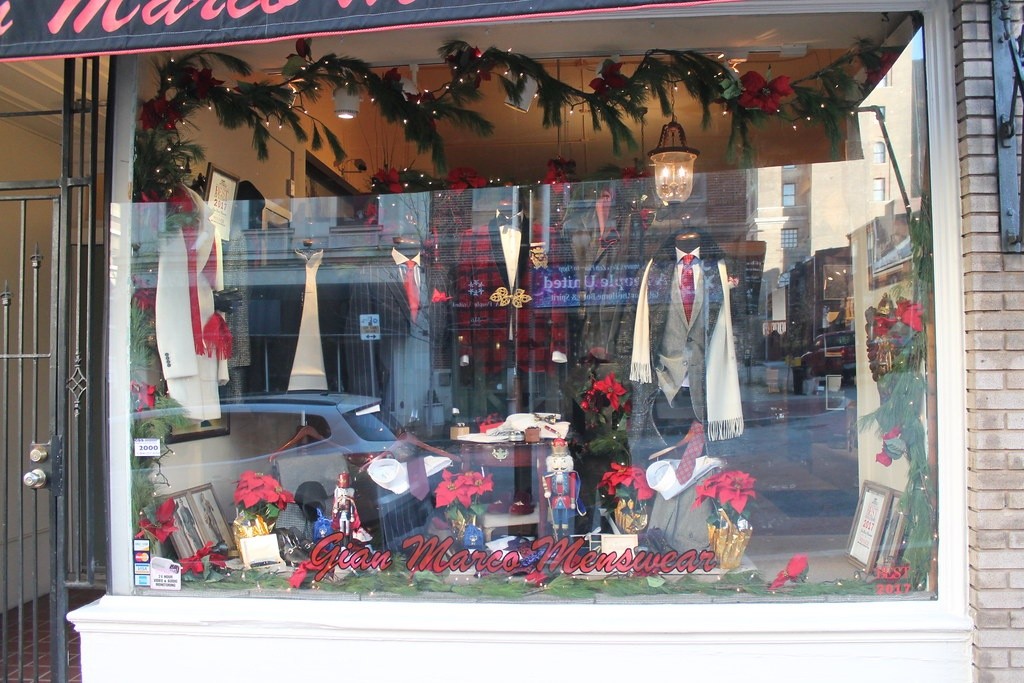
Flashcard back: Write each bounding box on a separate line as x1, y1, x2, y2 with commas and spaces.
648, 420, 705, 486
407, 456, 430, 501
405, 261, 420, 324
680, 255, 695, 323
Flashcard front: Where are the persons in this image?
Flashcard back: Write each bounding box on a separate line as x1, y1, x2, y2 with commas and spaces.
543, 439, 585, 546
455, 183, 568, 423
342, 226, 454, 439
244, 236, 349, 392
331, 473, 361, 553
618, 212, 743, 461
178, 497, 204, 551
201, 492, 223, 543
562, 180, 645, 371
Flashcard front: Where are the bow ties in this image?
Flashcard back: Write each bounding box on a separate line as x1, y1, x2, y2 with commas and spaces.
497, 213, 521, 232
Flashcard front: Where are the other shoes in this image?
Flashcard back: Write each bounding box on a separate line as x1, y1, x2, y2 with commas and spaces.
508, 503, 534, 516
487, 501, 509, 514
